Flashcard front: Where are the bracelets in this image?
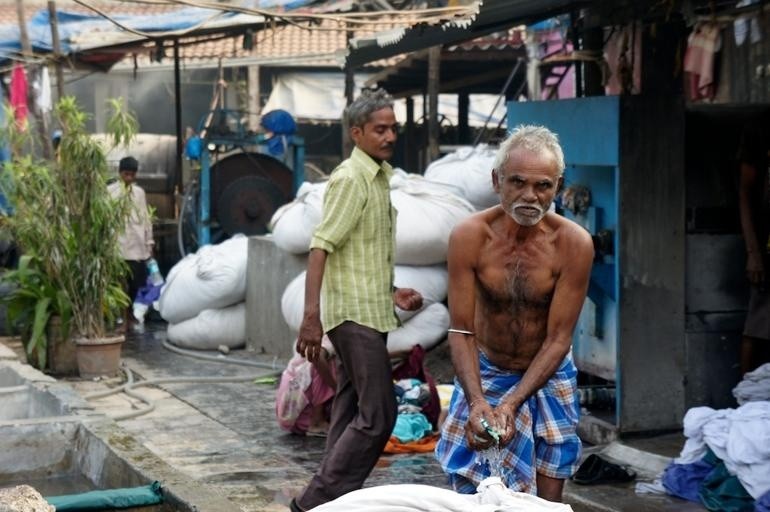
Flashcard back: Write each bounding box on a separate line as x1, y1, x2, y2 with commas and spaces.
444, 328, 476, 338
470, 399, 487, 406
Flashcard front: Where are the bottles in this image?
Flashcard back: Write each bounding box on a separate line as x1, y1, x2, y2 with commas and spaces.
147, 258, 164, 287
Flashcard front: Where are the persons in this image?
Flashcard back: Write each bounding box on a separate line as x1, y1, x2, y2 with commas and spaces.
436, 126, 596, 501
289, 87, 421, 510
105, 157, 156, 335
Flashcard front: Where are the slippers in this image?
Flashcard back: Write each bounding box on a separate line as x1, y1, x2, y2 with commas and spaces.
569, 453, 638, 485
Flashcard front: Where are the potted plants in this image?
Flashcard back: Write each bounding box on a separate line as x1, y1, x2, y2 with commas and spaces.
0, 93, 157, 380
